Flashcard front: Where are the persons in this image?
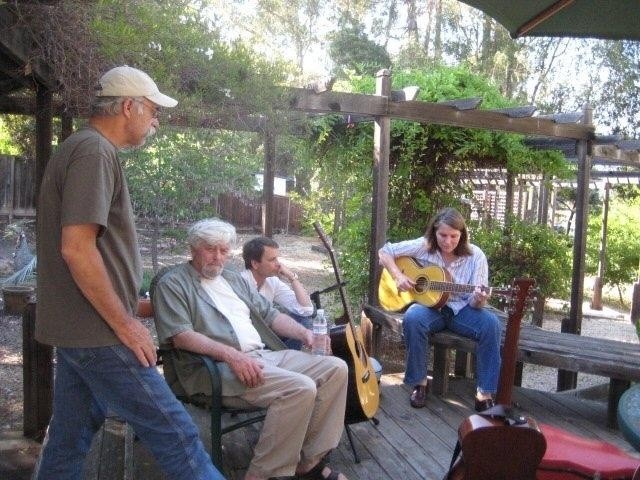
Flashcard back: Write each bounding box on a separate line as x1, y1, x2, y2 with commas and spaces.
150, 215, 357, 479
376, 206, 502, 413
33, 64, 229, 479
240, 235, 321, 352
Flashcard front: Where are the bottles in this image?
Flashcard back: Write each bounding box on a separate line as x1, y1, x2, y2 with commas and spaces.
559, 303, 570, 332
312, 309, 329, 356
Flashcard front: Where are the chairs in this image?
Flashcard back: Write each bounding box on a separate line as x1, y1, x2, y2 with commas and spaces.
148, 262, 267, 473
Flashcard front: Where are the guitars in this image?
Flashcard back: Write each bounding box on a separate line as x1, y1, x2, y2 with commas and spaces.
313, 221, 378, 420
378, 256, 540, 313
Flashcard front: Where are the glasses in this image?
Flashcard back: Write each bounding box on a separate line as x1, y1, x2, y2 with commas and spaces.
134, 99, 161, 118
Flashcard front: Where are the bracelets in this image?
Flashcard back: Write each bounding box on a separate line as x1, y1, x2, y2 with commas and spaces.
290, 272, 302, 285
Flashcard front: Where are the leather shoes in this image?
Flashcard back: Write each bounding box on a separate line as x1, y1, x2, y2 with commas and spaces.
411, 379, 428, 408
474, 395, 500, 418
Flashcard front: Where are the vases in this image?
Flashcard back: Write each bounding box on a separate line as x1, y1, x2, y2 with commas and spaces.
2, 285, 33, 315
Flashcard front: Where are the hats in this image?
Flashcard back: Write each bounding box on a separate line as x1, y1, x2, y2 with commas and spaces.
96, 64, 178, 107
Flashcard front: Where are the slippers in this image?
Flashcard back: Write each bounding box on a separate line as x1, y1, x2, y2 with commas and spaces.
296, 461, 340, 480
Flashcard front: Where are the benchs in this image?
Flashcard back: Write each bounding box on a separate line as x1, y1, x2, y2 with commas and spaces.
359, 296, 640, 428
